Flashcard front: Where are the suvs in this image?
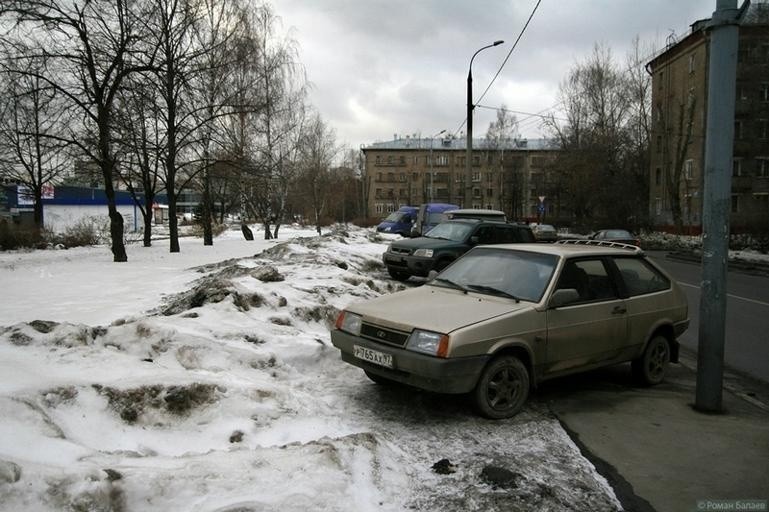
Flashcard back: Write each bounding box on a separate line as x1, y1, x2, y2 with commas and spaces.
376, 203, 536, 281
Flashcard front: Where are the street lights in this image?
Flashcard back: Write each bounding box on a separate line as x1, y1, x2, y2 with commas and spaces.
465, 40, 505, 208
430, 130, 447, 203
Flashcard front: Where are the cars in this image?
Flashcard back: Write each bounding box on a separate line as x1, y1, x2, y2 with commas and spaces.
588, 230, 641, 248
531, 225, 558, 244
329, 241, 690, 419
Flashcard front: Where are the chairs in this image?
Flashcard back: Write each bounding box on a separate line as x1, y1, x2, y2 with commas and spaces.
604, 268, 640, 297
